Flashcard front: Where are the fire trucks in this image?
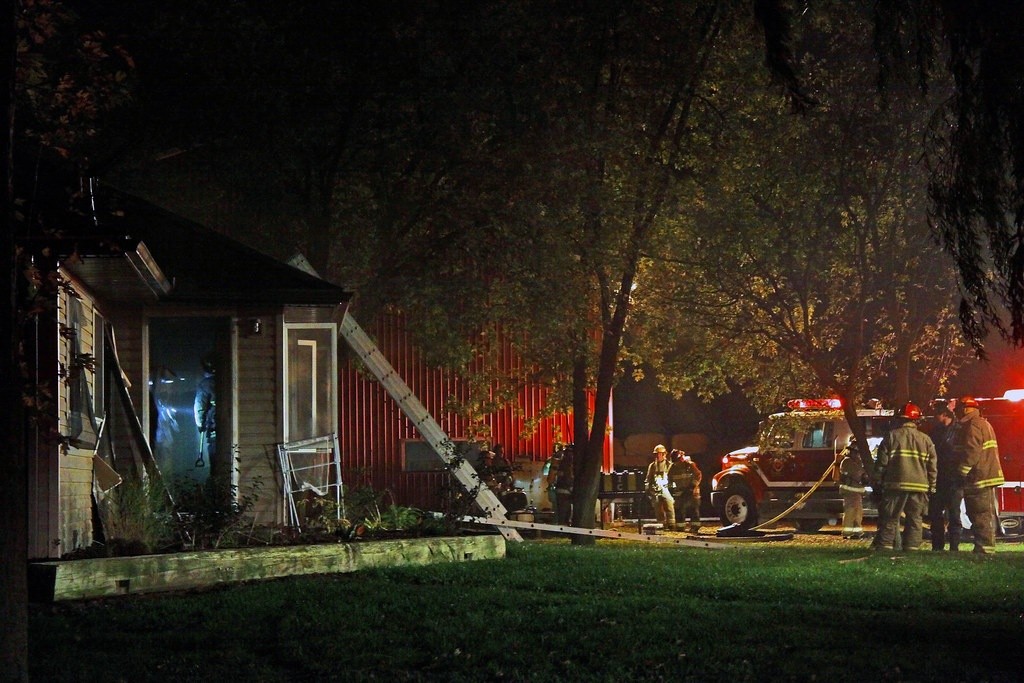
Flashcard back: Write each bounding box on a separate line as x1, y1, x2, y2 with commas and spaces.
710, 389, 1023, 538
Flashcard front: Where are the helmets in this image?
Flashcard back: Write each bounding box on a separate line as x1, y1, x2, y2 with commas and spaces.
846, 440, 859, 451
929, 398, 949, 414
477, 451, 494, 460
896, 402, 921, 418
953, 394, 980, 411
653, 444, 665, 453
670, 450, 681, 461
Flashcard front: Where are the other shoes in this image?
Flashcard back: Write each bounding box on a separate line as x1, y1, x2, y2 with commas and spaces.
843, 534, 859, 539
861, 533, 874, 537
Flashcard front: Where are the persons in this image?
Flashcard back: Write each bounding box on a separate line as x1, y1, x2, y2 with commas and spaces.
931, 399, 964, 551
954, 397, 1004, 554
669, 449, 701, 532
645, 444, 676, 530
872, 402, 937, 551
474, 451, 495, 480
543, 442, 574, 526
493, 445, 514, 490
839, 433, 868, 538
194, 356, 216, 478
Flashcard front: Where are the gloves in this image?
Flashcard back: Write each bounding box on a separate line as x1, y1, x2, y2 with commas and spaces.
927, 486, 936, 496
